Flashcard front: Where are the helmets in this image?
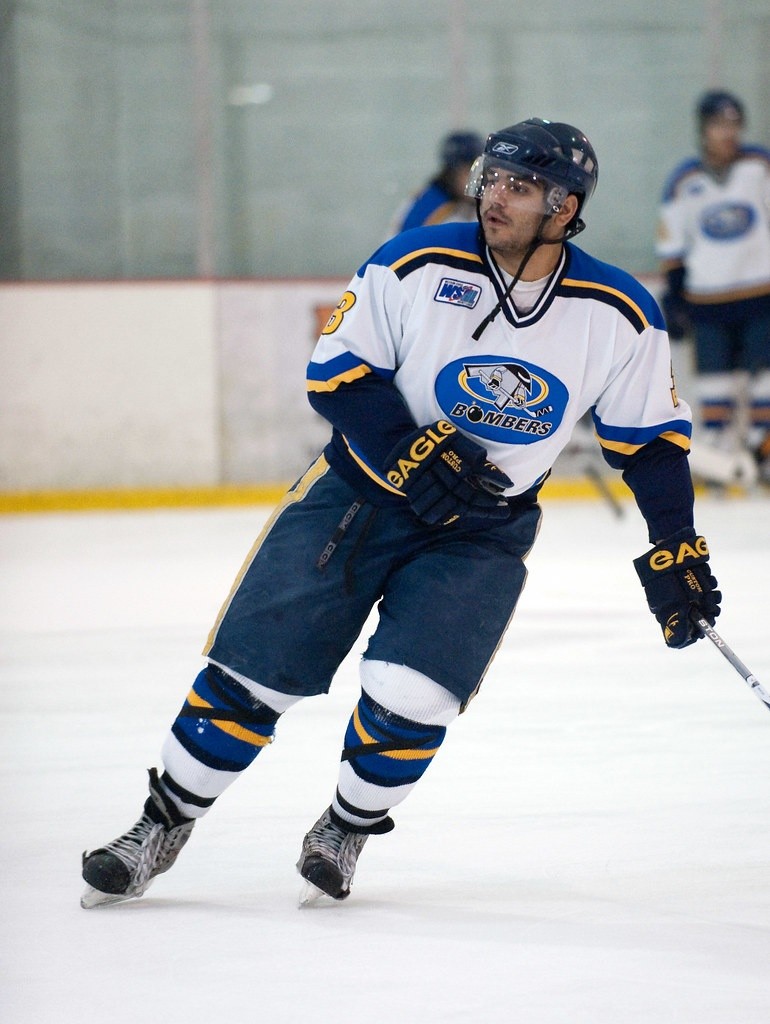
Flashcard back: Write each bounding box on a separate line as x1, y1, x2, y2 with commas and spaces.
463, 116, 600, 234
444, 133, 487, 175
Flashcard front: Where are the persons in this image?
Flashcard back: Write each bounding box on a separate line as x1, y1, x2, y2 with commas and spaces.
394, 128, 489, 237
80, 118, 721, 910
659, 89, 770, 487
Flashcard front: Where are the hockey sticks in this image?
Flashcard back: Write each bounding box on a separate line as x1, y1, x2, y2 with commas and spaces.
564, 436, 629, 518
694, 613, 770, 710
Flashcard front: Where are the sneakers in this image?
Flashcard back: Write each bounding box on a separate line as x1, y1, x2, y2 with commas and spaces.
79, 767, 196, 910
296, 805, 370, 907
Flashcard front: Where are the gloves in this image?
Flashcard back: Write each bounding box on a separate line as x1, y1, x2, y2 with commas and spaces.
634, 527, 721, 651
380, 419, 514, 542
660, 284, 691, 339
